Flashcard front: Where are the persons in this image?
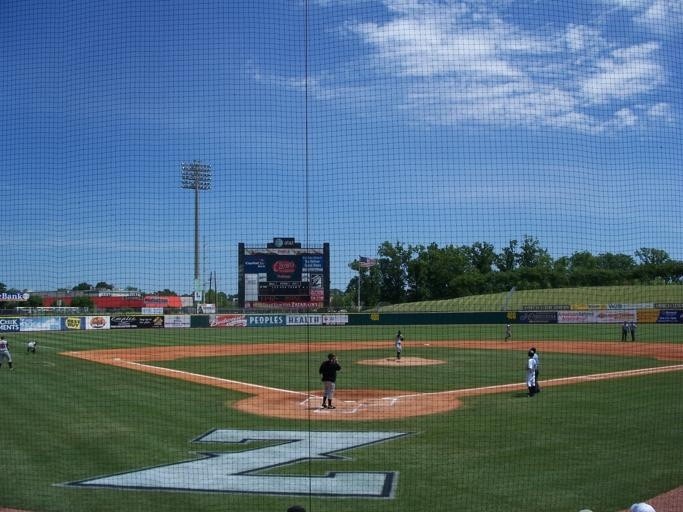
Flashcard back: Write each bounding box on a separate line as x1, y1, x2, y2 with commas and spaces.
395, 334, 402, 360
25, 339, 36, 354
394, 330, 404, 345
528, 347, 539, 394
525, 350, 537, 397
319, 352, 340, 410
504, 321, 512, 342
629, 320, 637, 342
0, 335, 13, 371
619, 321, 629, 342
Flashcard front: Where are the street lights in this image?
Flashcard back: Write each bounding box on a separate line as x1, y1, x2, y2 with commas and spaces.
179, 157, 213, 313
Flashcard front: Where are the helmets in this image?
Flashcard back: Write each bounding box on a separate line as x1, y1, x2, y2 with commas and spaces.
528, 348, 536, 356
328, 353, 336, 359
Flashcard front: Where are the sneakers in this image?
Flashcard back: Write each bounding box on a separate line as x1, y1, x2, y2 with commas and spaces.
322, 403, 336, 409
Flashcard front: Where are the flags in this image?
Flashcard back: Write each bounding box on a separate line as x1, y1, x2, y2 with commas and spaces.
358, 255, 377, 269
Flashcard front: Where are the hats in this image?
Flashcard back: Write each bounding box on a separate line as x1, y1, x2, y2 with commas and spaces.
631, 503, 656, 512
